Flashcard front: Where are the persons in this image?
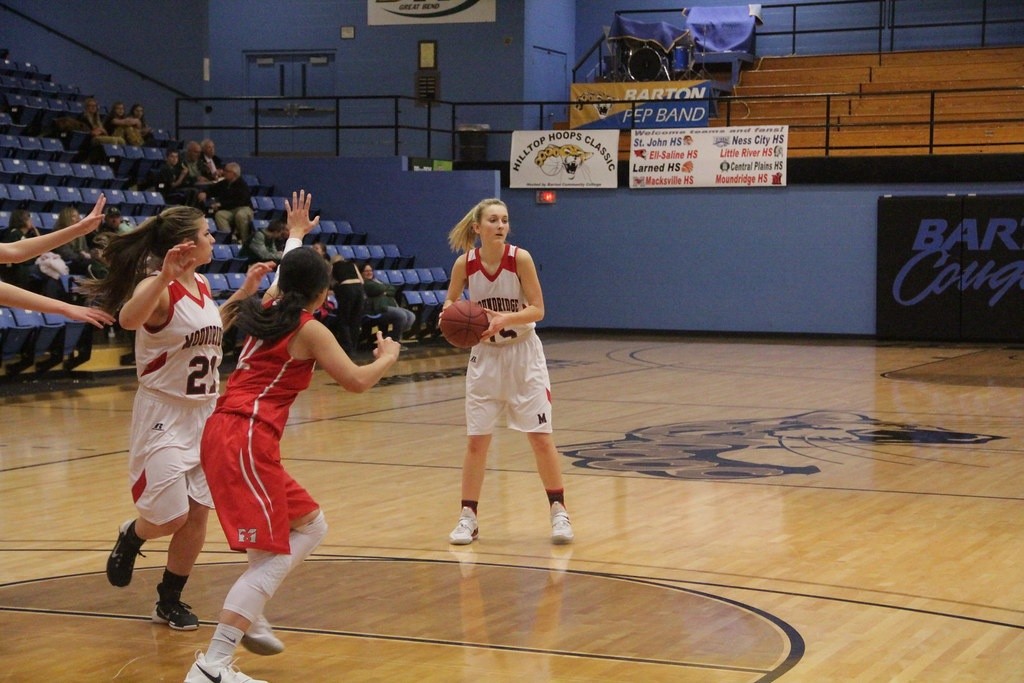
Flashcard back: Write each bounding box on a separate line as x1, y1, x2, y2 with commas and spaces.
181, 189, 401, 683
437, 199, 574, 545
145, 140, 416, 354
76, 98, 154, 151
72, 206, 275, 631
0, 193, 116, 329
0, 207, 130, 305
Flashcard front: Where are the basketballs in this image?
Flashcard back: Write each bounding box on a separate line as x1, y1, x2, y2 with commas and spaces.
441, 302, 488, 348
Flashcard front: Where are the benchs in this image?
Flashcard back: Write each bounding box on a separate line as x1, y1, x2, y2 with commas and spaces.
549, 45, 1024, 160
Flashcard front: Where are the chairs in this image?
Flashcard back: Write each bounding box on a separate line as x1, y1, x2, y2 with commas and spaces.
0, 46, 471, 375
683, 3, 764, 82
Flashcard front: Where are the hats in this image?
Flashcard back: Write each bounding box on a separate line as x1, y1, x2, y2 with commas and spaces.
105, 206, 120, 216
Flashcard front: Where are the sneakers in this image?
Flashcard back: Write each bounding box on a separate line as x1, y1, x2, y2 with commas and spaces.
450, 507, 478, 545
107, 519, 146, 588
551, 502, 572, 541
183, 653, 270, 683
152, 582, 200, 631
242, 615, 283, 655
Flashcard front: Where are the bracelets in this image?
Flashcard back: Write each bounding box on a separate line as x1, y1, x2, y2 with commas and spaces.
239, 286, 251, 299
